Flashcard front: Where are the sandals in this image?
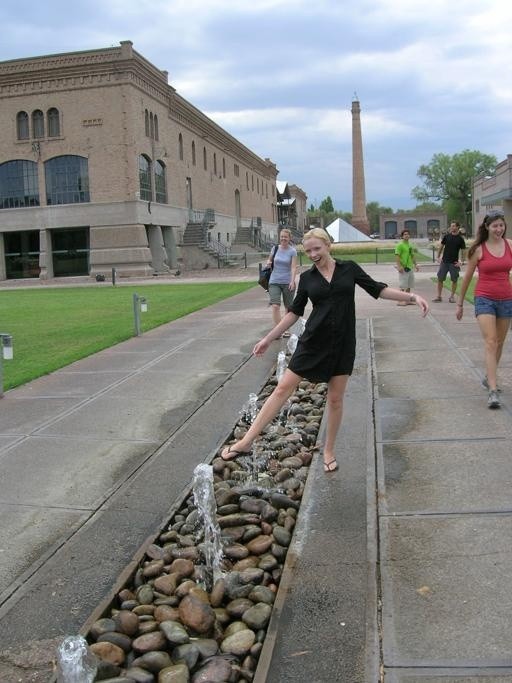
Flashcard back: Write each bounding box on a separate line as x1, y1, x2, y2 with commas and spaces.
283, 330, 291, 338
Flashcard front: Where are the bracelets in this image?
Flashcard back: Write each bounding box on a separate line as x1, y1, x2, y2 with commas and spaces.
410, 293, 417, 302
457, 303, 463, 307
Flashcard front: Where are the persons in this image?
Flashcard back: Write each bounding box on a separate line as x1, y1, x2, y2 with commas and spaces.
267, 229, 297, 339
221, 228, 429, 473
432, 220, 466, 303
395, 230, 419, 305
456, 210, 512, 408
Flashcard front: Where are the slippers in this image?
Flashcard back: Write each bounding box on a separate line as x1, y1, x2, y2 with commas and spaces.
323, 459, 338, 473
220, 446, 253, 461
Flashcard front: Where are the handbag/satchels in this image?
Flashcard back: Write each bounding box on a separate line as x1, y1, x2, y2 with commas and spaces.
258, 264, 272, 292
404, 267, 411, 272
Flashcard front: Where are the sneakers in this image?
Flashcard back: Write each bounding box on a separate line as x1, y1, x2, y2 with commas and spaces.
432, 297, 441, 303
448, 297, 456, 303
482, 375, 504, 409
